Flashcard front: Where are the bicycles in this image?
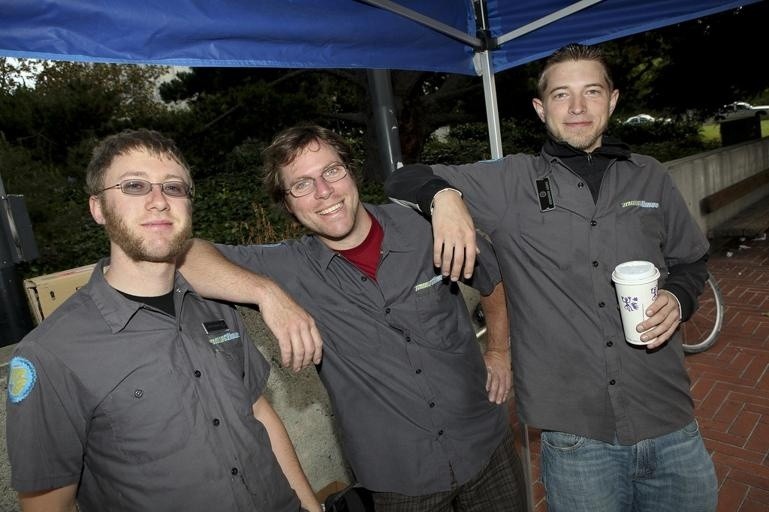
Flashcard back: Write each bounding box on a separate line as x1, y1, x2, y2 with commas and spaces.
681, 268, 725, 354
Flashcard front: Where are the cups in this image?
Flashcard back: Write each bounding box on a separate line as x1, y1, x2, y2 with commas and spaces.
611, 261, 660, 345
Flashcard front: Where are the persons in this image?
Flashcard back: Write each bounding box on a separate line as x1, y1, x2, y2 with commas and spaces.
382, 42, 721, 510
5, 127, 329, 512
178, 125, 532, 510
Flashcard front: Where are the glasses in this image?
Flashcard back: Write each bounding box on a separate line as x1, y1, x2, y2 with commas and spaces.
93, 179, 190, 197
284, 162, 347, 198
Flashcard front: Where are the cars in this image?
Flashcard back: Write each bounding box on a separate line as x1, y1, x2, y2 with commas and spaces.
623, 114, 671, 124
706, 101, 769, 124
